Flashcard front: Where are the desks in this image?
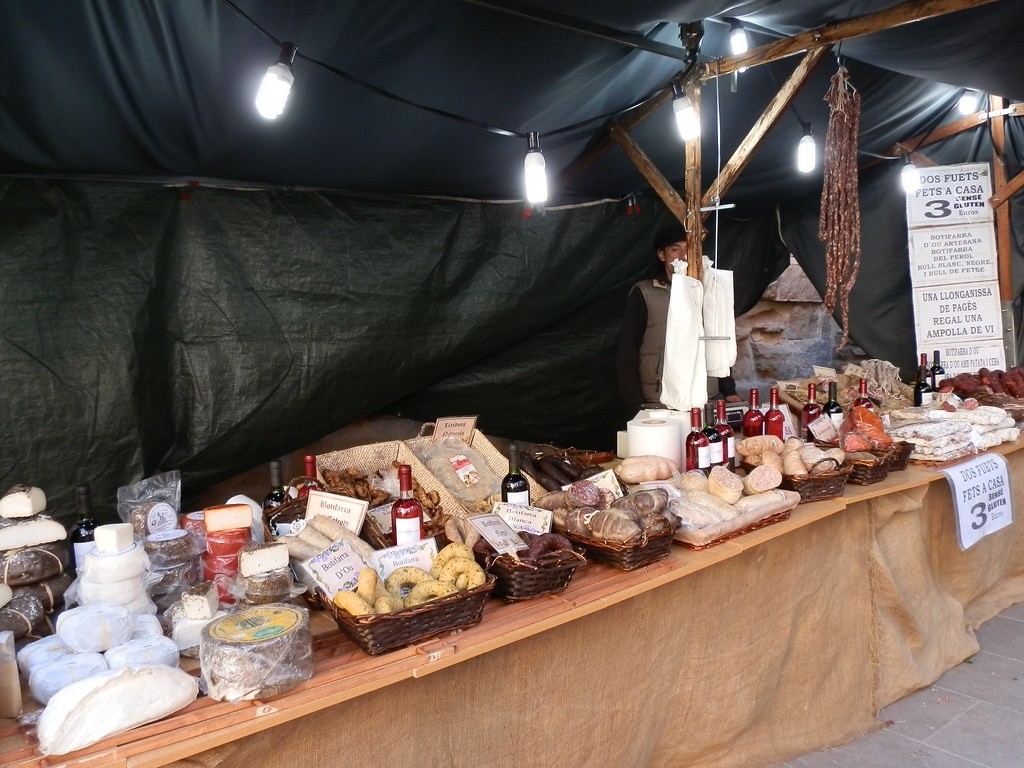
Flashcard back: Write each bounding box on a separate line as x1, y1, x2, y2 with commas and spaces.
1, 413, 1023, 767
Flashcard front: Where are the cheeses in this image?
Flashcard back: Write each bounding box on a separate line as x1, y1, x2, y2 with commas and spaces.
0, 482, 293, 755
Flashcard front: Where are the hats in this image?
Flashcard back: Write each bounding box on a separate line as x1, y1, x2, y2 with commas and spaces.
654, 221, 686, 252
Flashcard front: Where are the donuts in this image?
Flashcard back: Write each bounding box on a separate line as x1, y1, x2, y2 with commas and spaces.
333, 517, 487, 616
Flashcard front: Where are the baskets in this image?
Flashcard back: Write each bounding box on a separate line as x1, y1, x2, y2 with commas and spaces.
262, 476, 389, 610
315, 441, 466, 548
739, 457, 854, 505
472, 547, 584, 602
404, 421, 547, 517
553, 516, 683, 572
314, 567, 498, 655
813, 439, 916, 486
968, 391, 1024, 420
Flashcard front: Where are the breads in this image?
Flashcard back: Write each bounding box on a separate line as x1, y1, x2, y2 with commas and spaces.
277, 515, 375, 561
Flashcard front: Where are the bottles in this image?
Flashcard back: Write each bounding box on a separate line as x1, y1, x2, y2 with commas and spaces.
391, 464, 424, 546
854, 377, 874, 414
70, 485, 98, 576
685, 407, 712, 478
920, 353, 933, 390
765, 388, 786, 444
715, 400, 736, 474
822, 382, 844, 431
930, 350, 946, 393
500, 441, 531, 506
262, 460, 292, 536
742, 388, 764, 440
801, 384, 822, 443
703, 403, 724, 469
298, 454, 321, 498
913, 365, 933, 407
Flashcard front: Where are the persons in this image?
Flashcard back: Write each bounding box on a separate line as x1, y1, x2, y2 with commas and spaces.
615, 224, 741, 421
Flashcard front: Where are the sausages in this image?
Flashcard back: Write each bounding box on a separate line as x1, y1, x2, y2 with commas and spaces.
472, 531, 573, 558
888, 407, 1018, 459
519, 451, 605, 492
819, 93, 861, 352
939, 367, 1024, 398
